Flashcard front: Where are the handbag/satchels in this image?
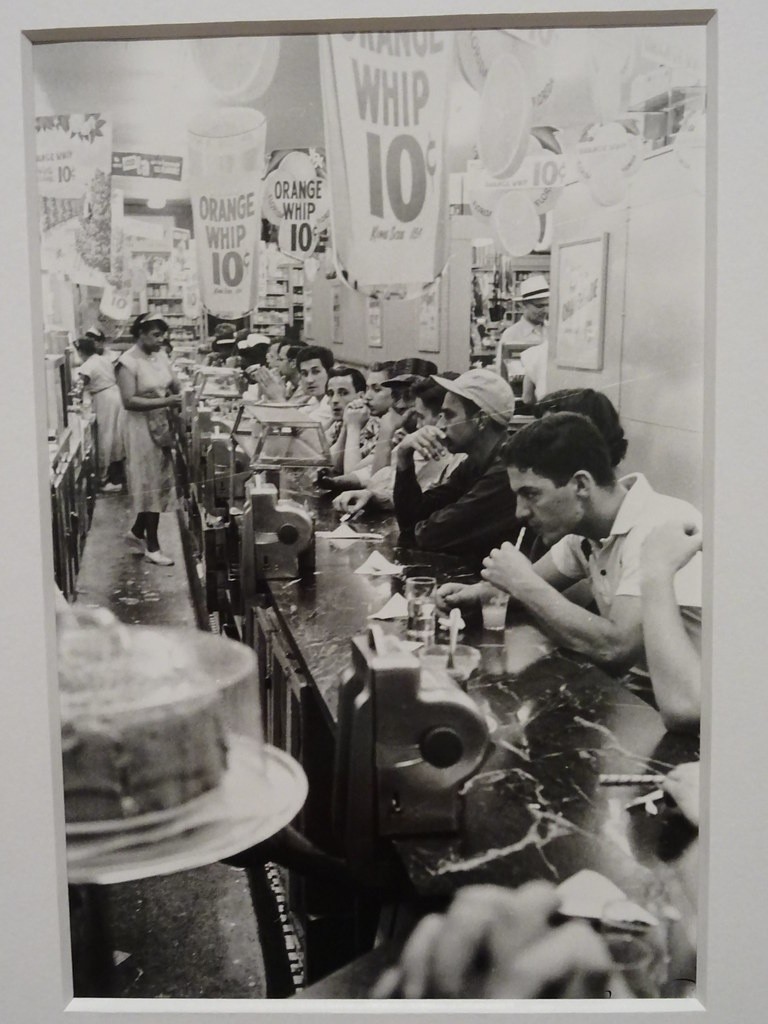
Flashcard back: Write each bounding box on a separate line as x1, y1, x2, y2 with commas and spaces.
147, 406, 181, 447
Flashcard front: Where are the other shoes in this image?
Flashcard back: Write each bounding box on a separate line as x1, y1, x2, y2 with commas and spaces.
126, 530, 148, 546
98, 482, 122, 491
144, 548, 174, 566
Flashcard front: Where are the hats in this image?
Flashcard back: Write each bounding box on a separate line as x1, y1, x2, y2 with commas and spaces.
512, 275, 550, 301
379, 358, 438, 387
430, 368, 515, 425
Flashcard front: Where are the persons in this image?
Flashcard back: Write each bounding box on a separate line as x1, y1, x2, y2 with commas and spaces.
72, 317, 702, 828
111, 311, 186, 567
372, 872, 613, 1000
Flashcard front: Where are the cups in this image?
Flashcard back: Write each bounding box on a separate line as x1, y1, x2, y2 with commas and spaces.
479, 587, 510, 629
601, 934, 653, 999
407, 577, 436, 637
601, 900, 667, 991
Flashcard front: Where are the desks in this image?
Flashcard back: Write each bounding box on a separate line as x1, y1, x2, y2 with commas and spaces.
229, 492, 699, 915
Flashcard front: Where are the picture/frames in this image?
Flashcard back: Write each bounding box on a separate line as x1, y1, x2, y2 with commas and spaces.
553, 231, 609, 372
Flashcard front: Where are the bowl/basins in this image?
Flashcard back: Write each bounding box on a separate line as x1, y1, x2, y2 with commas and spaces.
419, 644, 480, 681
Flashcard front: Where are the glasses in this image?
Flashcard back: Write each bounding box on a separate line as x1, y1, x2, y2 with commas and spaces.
530, 303, 549, 308
